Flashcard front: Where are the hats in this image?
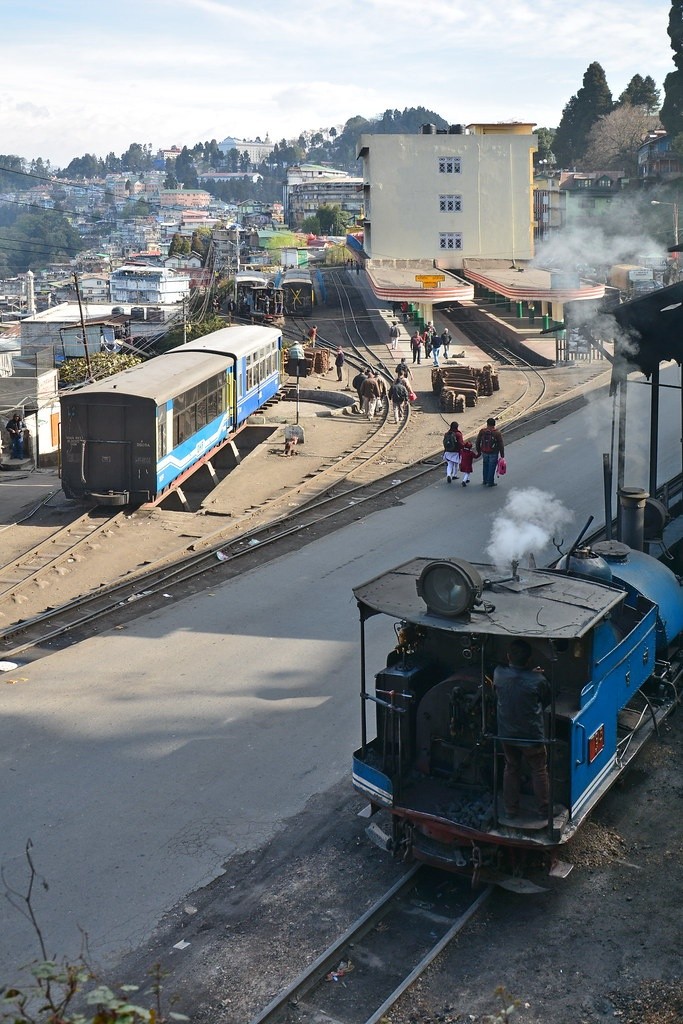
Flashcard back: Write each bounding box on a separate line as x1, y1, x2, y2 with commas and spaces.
465, 441, 472, 449
433, 332, 438, 335
503, 639, 533, 665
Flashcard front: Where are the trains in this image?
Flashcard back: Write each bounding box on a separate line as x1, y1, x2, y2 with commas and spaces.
250, 282, 283, 326
280, 267, 315, 317
234, 270, 266, 315
59, 324, 281, 506
352, 280, 682, 898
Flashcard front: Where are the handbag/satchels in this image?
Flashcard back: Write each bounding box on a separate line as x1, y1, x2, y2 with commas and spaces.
410, 393, 418, 401
395, 392, 404, 405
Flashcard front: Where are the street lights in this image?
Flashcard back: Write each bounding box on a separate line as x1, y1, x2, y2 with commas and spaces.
228, 228, 245, 269
650, 198, 678, 266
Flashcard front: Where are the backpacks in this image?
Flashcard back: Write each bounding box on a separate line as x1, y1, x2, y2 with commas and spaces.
496, 457, 507, 478
443, 431, 458, 451
481, 429, 498, 453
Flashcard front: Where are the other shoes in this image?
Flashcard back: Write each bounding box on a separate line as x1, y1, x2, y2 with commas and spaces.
367, 414, 373, 420
537, 806, 563, 820
399, 416, 405, 421
503, 813, 517, 820
394, 420, 399, 424
483, 482, 497, 487
466, 480, 470, 483
462, 481, 466, 487
452, 477, 459, 479
447, 476, 451, 483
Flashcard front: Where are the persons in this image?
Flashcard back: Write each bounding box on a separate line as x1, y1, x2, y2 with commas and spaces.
353, 302, 452, 424
307, 325, 318, 347
257, 291, 284, 315
355, 260, 359, 275
288, 340, 305, 360
443, 418, 505, 487
335, 345, 345, 381
491, 639, 563, 819
5, 413, 28, 460
